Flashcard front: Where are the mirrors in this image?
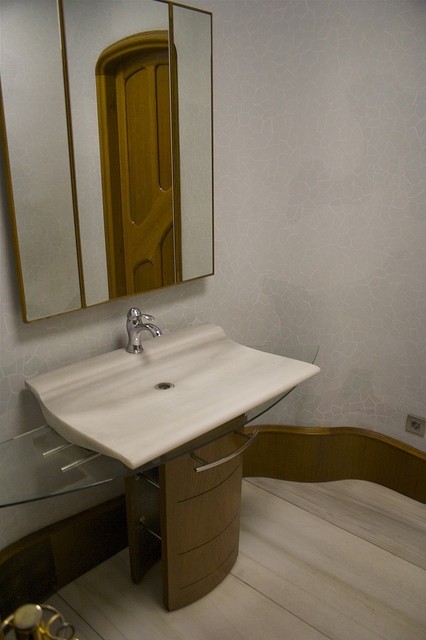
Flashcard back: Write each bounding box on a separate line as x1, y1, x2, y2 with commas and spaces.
2, 1, 214, 324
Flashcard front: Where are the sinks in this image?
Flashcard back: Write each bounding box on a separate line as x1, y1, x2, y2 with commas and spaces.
25, 322, 320, 464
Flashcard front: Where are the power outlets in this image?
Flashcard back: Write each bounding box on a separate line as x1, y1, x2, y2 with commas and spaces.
405, 414, 426, 437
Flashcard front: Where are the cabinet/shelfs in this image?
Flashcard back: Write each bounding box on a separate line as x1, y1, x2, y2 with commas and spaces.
124, 412, 260, 612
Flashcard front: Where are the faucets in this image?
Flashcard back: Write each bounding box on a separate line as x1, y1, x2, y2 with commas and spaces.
126, 307, 161, 355
